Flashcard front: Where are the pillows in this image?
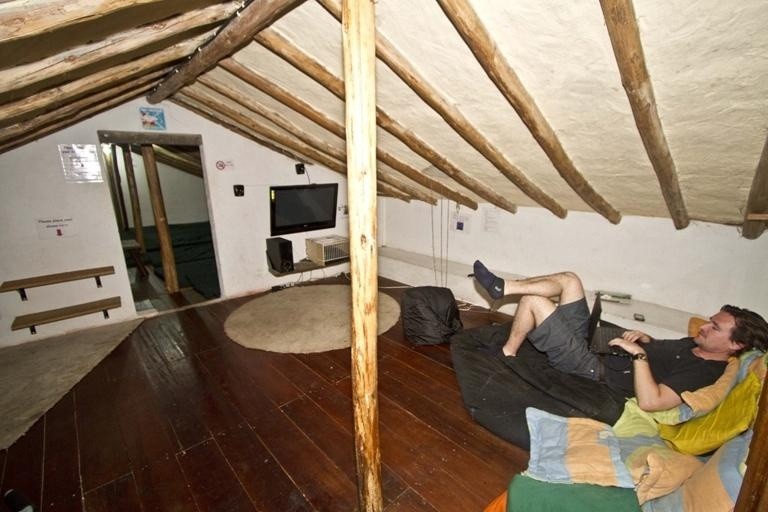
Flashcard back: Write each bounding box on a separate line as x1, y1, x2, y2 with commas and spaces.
659, 372, 759, 453
738, 347, 768, 383
526, 406, 633, 487
613, 397, 701, 504
641, 413, 753, 512
626, 358, 737, 425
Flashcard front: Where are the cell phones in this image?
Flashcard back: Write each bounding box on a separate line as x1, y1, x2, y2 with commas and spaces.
633, 313, 646, 321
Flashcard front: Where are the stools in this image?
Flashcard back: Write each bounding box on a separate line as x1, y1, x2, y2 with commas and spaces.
120, 240, 149, 278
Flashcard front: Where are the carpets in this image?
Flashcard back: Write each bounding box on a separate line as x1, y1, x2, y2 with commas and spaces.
223, 284, 401, 354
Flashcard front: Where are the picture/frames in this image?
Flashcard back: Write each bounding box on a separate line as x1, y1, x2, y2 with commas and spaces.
140, 108, 166, 131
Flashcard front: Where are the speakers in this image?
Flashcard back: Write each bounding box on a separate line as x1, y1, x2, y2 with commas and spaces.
266, 236, 294, 273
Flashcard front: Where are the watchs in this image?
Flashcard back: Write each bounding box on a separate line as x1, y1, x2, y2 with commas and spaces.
632, 354, 649, 362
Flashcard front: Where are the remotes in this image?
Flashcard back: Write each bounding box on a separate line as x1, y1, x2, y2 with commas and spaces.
594, 291, 631, 300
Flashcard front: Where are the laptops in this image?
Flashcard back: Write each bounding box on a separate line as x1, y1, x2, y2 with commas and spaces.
588, 292, 636, 357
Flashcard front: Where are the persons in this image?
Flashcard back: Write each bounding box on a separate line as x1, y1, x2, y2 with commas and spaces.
474, 259, 767, 412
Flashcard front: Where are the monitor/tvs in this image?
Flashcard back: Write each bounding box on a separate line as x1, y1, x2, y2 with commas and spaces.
270, 183, 339, 235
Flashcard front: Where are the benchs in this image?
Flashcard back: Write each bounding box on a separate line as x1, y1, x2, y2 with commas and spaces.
0, 266, 116, 301
12, 296, 124, 334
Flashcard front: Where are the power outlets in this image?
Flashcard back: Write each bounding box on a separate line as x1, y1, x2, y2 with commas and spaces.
296, 164, 305, 175
300, 259, 311, 261
